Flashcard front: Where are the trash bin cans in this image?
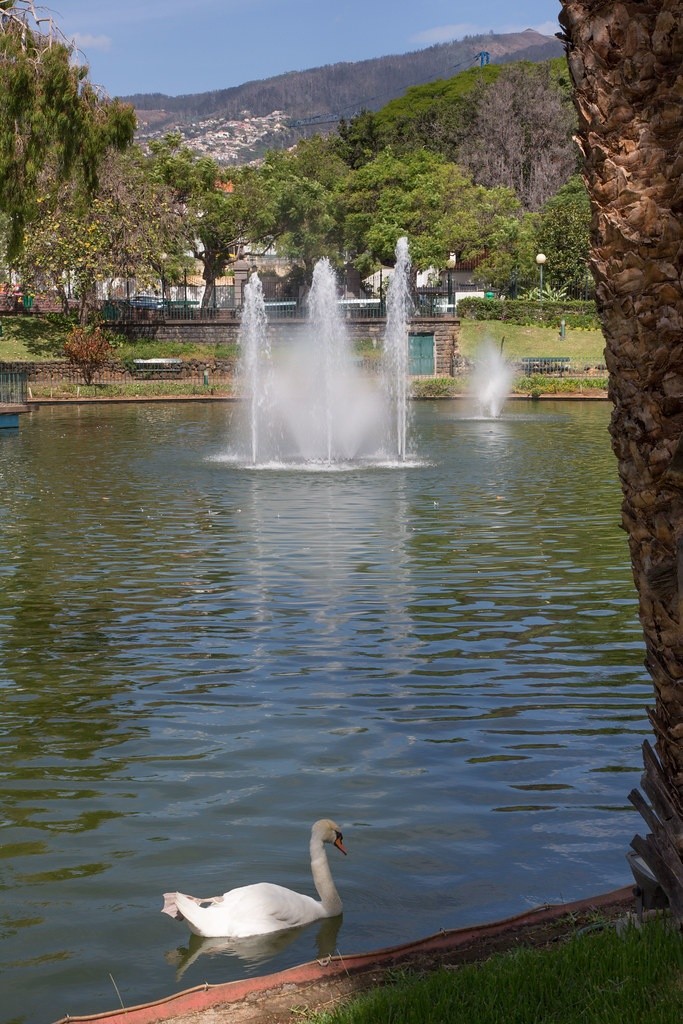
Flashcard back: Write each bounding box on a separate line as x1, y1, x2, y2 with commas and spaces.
103, 300, 134, 319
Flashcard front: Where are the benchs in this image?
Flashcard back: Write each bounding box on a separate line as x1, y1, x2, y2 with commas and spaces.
131, 359, 183, 380
522, 357, 570, 378
161, 300, 199, 304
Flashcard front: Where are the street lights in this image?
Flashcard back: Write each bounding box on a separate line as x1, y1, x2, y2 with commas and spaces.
536, 253, 547, 300
446, 249, 456, 311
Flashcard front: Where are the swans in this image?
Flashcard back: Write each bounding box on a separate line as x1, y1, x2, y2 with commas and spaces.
160, 819, 350, 978
